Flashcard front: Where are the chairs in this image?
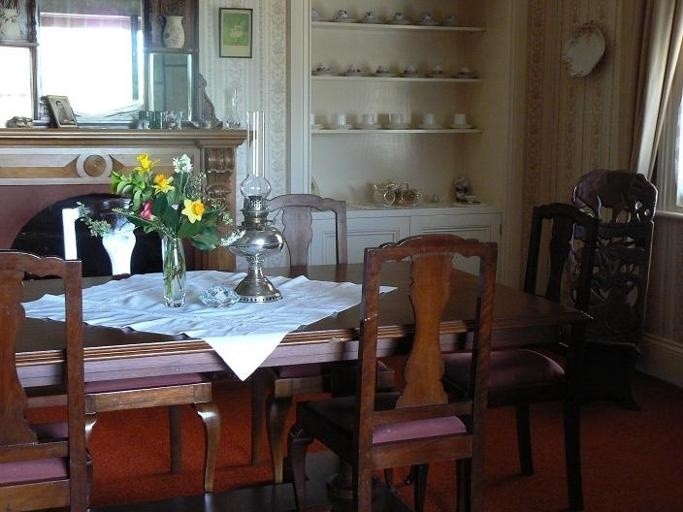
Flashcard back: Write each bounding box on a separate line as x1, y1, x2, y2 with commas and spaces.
552, 169, 659, 412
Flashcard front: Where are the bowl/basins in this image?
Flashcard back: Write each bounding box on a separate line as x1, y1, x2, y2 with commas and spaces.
310, 6, 463, 19
313, 62, 472, 72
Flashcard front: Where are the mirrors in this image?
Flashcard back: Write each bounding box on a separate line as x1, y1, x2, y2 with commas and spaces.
28, 0, 149, 127
146, 49, 200, 129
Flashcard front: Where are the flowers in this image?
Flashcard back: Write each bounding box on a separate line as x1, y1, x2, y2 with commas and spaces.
76, 152, 247, 301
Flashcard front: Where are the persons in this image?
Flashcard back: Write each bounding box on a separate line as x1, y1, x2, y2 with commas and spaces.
55, 100, 74, 126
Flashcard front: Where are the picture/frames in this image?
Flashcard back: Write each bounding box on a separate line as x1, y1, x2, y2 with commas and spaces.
217, 7, 253, 59
0, 0, 40, 47
45, 95, 79, 127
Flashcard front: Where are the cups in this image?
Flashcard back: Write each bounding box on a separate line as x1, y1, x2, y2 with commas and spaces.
136, 108, 186, 131
311, 112, 467, 126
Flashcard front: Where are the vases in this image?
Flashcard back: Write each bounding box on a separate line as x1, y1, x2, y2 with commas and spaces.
162, 15, 185, 48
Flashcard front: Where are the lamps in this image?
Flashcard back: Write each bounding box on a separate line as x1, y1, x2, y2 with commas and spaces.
227, 112, 285, 303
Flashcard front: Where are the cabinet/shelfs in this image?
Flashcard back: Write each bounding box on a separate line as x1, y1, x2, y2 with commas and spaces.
285, 1, 518, 211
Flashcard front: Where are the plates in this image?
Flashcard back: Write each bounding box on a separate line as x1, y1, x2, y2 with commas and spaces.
312, 73, 480, 77
314, 123, 475, 132
310, 21, 469, 23
